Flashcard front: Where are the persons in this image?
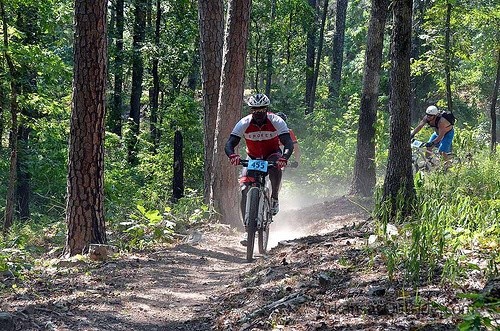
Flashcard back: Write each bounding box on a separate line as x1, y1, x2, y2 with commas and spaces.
410, 106, 456, 172
274, 112, 299, 195
224, 94, 294, 247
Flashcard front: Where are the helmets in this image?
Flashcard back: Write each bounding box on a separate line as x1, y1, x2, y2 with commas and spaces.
426, 106, 438, 115
275, 112, 286, 122
248, 93, 270, 107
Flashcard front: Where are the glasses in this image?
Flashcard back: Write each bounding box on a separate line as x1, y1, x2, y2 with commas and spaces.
250, 107, 268, 114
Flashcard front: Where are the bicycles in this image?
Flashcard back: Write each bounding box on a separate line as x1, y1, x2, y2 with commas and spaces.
411, 138, 460, 177
233, 159, 291, 263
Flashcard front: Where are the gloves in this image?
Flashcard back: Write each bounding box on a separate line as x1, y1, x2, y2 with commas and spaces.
289, 161, 298, 168
229, 154, 241, 166
426, 141, 433, 147
274, 157, 287, 171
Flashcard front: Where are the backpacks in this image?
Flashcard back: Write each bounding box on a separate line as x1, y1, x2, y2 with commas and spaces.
426, 107, 458, 131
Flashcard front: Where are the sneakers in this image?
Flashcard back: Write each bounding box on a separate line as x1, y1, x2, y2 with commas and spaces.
240, 232, 248, 245
271, 194, 279, 215
